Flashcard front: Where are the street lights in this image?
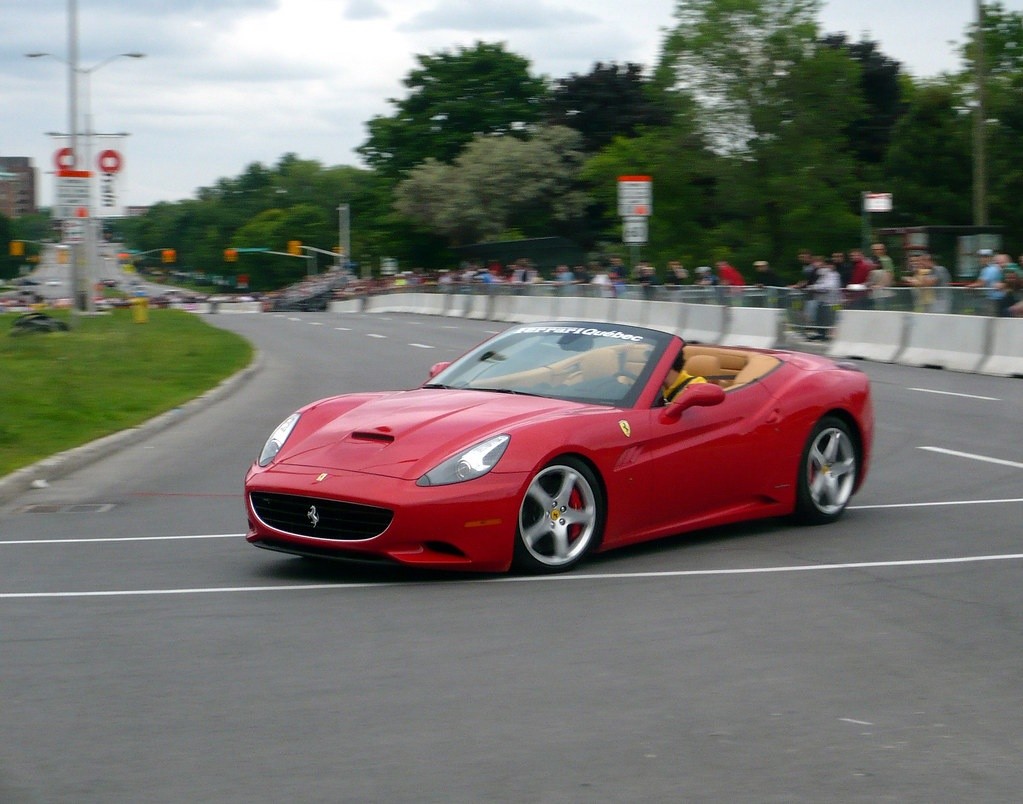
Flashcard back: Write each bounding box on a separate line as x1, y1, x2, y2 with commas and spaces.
22, 51, 151, 177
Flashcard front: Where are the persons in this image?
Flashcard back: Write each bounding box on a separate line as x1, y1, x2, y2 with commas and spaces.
646, 349, 707, 406
394, 243, 1023, 341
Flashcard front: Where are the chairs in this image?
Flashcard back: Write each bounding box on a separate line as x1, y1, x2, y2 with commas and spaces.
684, 354, 719, 389
571, 349, 628, 385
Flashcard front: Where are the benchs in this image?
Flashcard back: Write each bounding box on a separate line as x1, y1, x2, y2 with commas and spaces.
625, 343, 780, 391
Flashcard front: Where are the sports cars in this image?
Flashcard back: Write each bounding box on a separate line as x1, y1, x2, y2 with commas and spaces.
243, 322, 876, 576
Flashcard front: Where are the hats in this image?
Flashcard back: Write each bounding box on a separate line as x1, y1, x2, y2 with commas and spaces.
976, 249, 993, 256
